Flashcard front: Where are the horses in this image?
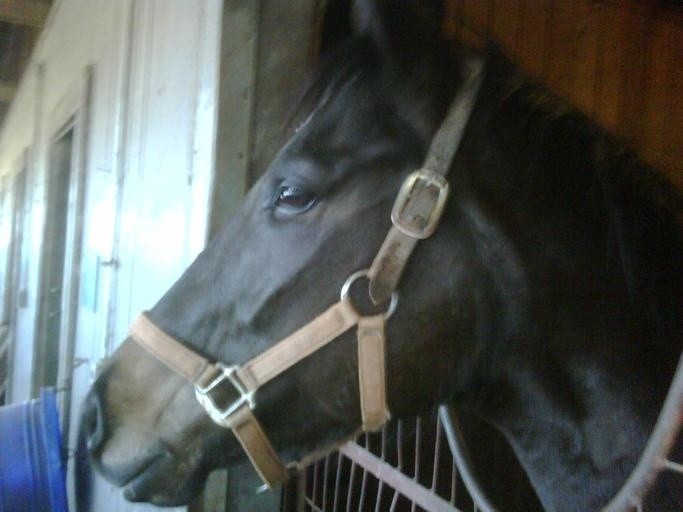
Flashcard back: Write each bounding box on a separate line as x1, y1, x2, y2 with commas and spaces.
79, 1, 683, 512
